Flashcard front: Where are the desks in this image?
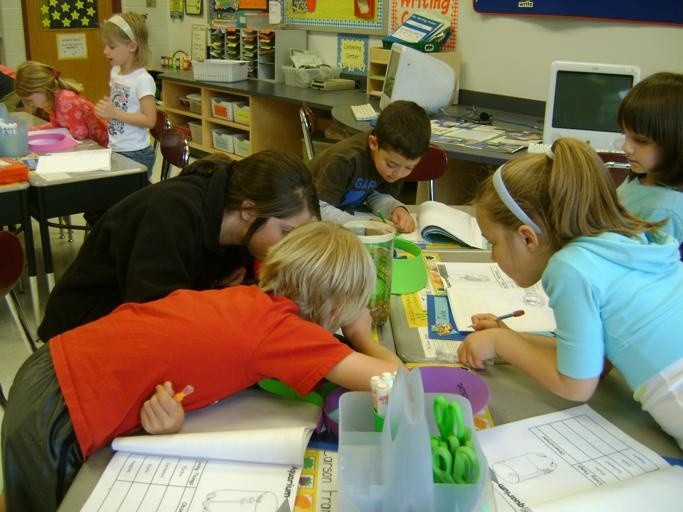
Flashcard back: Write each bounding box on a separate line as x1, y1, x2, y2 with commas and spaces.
253, 79, 545, 207
0, 109, 683, 512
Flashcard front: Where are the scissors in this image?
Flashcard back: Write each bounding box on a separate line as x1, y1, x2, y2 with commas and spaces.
429, 395, 479, 485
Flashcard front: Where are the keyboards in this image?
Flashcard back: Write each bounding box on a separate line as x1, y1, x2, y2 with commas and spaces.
350, 103, 375, 121
528, 143, 624, 154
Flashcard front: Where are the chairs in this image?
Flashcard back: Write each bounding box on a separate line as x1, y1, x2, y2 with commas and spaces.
296, 100, 320, 165
587, 148, 633, 189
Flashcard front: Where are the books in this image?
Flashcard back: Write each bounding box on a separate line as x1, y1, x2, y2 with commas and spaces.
475, 401, 683, 512
81, 388, 322, 512
353, 201, 489, 250
430, 118, 543, 154
436, 262, 558, 334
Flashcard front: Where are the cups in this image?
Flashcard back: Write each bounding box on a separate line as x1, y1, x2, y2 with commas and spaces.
0, 118, 28, 157
338, 219, 395, 326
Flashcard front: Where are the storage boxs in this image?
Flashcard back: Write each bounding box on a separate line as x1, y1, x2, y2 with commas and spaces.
181, 91, 253, 158
280, 65, 344, 90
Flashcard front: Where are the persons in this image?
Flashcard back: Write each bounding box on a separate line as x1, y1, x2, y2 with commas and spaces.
37, 149, 321, 343
15, 61, 109, 147
307, 100, 431, 236
616, 72, 683, 247
0, 222, 411, 512
457, 138, 683, 453
0, 64, 21, 113
83, 11, 157, 227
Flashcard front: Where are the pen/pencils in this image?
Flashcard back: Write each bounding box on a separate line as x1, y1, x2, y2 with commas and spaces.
377, 210, 399, 236
370, 372, 393, 415
467, 310, 524, 327
171, 385, 194, 400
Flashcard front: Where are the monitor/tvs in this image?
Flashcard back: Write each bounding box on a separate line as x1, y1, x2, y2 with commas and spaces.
543, 61, 640, 155
379, 42, 456, 114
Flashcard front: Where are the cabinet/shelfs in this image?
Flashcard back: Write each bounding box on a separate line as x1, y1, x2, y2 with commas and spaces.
362, 45, 461, 110
144, 66, 298, 175
206, 22, 309, 85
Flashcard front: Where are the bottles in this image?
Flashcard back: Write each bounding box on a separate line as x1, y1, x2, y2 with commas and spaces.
160, 56, 190, 71
